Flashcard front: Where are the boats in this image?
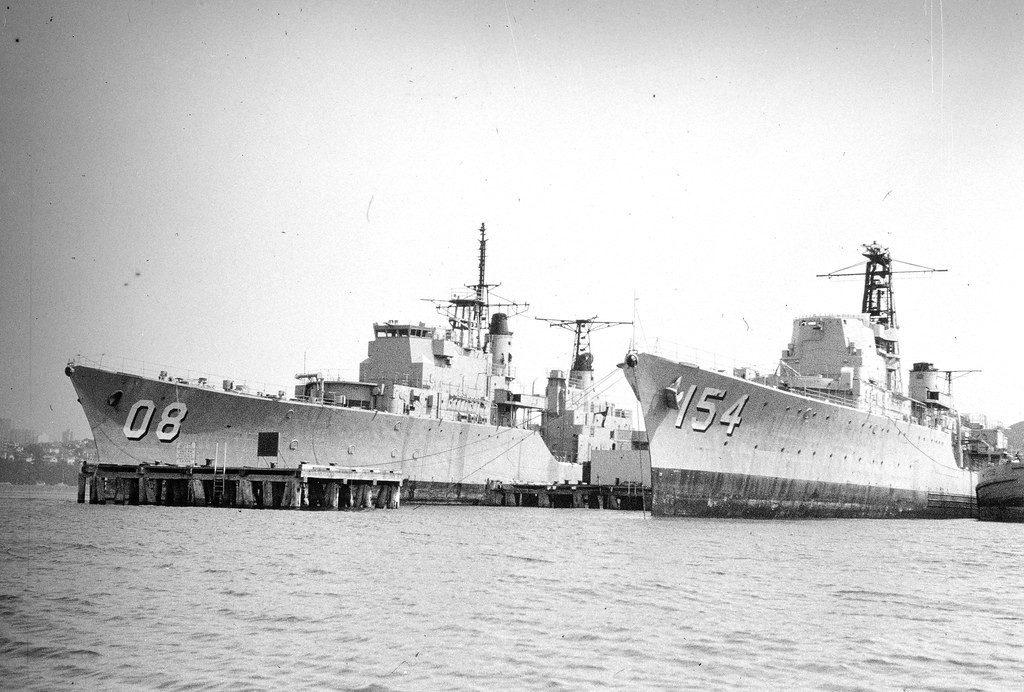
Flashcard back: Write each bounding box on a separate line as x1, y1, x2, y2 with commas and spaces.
65, 217, 585, 504
621, 242, 997, 520
